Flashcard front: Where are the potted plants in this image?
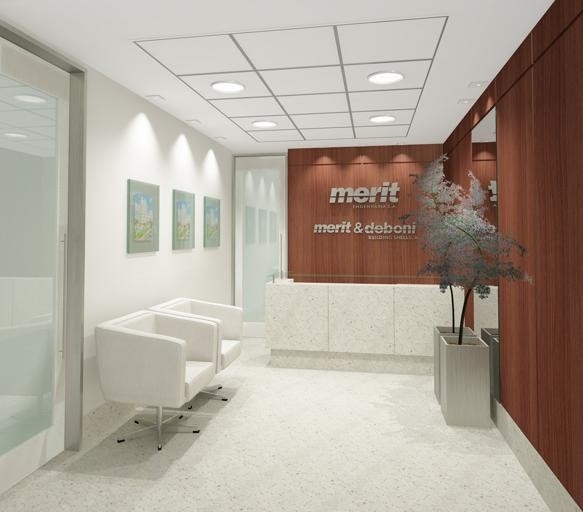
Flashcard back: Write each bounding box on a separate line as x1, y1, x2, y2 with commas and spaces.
437, 212, 535, 428
399, 152, 490, 404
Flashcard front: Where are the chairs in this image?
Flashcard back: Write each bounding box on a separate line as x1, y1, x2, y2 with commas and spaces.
149, 297, 243, 409
95, 309, 217, 451
